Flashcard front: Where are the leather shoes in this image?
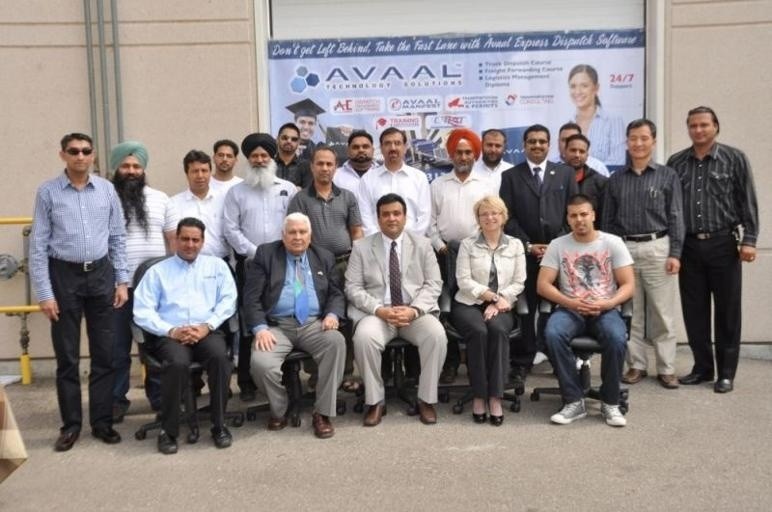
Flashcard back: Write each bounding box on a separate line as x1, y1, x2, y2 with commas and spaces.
158, 432, 177, 454
417, 402, 436, 423
55, 421, 120, 451
211, 426, 231, 446
621, 368, 732, 393
471, 413, 503, 425
269, 415, 286, 430
312, 413, 333, 438
364, 404, 386, 426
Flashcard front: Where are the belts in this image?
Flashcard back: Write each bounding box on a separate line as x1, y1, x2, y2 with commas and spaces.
622, 230, 665, 242
48, 255, 107, 272
694, 232, 727, 240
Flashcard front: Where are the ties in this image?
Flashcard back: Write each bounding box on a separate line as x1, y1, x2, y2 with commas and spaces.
294, 256, 310, 326
533, 168, 541, 192
389, 241, 403, 307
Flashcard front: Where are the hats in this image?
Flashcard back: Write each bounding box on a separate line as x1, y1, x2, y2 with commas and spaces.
286, 99, 325, 119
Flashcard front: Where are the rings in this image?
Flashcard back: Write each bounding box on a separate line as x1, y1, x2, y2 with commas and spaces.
750, 256, 754, 260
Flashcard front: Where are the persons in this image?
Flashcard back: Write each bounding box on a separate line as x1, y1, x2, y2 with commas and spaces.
286, 99, 326, 159
547, 64, 627, 165
600, 119, 686, 389
133, 218, 240, 454
29, 135, 130, 452
666, 106, 759, 394
110, 141, 177, 424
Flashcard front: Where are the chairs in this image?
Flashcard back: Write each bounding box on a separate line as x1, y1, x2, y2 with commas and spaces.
346, 248, 447, 418
528, 252, 633, 415
127, 256, 244, 446
233, 262, 346, 429
440, 240, 529, 414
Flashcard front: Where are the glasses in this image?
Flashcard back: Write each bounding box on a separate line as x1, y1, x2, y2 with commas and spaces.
526, 139, 547, 144
69, 148, 92, 155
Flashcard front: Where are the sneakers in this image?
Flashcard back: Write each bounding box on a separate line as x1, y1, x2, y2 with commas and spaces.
550, 401, 626, 426
